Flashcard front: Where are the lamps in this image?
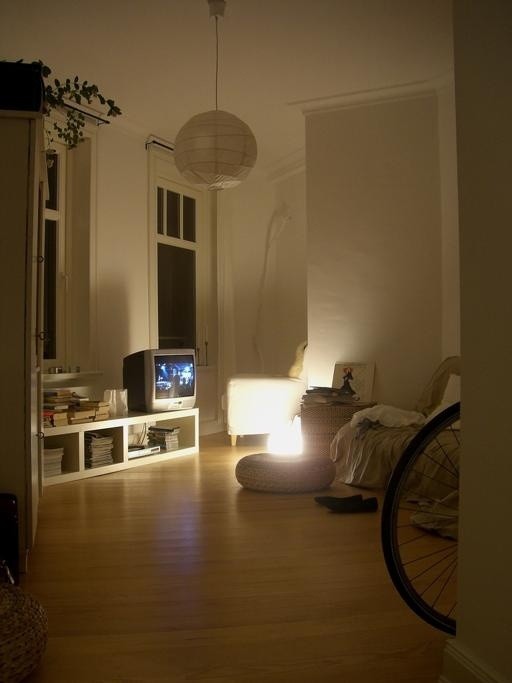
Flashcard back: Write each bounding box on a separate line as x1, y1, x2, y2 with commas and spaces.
169, 0, 259, 191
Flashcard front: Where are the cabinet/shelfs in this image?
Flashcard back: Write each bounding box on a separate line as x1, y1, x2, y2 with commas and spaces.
37, 408, 201, 488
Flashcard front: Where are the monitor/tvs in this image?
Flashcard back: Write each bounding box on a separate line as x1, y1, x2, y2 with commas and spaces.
122, 348, 197, 413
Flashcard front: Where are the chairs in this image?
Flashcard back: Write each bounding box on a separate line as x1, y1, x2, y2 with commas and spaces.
331, 357, 462, 508
221, 344, 309, 449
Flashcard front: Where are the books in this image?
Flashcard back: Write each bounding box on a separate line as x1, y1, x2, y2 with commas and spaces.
301, 385, 353, 411
85, 433, 114, 469
43, 389, 112, 428
44, 448, 64, 477
128, 444, 145, 452
146, 426, 181, 453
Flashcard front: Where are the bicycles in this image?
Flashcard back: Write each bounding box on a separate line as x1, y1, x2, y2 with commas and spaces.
381, 401, 460, 636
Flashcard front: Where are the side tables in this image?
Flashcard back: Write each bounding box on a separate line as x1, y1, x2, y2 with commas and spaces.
301, 402, 377, 458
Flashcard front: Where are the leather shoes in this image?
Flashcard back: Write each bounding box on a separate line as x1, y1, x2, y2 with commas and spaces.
314, 494, 378, 513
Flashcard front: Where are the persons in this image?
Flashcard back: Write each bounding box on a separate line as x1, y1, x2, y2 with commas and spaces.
155, 362, 193, 397
341, 368, 360, 401
